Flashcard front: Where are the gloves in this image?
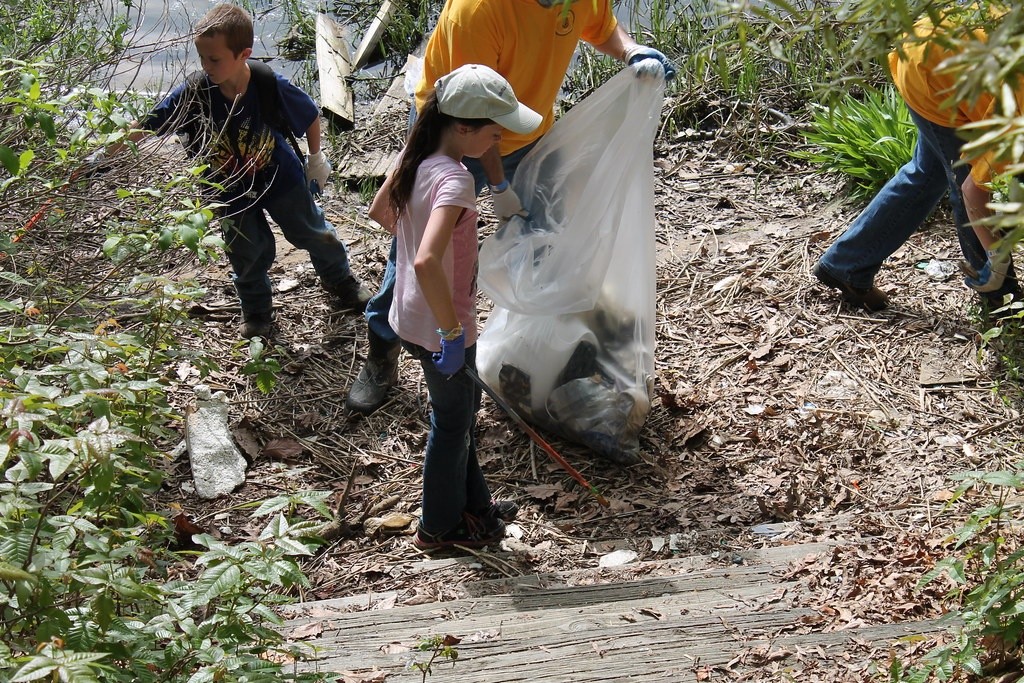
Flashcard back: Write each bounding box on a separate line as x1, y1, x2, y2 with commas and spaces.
433, 324, 465, 375
307, 149, 331, 195
627, 43, 676, 81
963, 253, 1010, 293
486, 182, 522, 221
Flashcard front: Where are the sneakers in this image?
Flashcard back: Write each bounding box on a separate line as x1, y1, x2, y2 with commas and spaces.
814, 253, 888, 312
323, 278, 372, 311
416, 499, 520, 551
239, 313, 275, 339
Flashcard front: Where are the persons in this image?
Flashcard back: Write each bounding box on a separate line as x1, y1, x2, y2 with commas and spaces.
368, 63, 544, 550
348, 0, 675, 411
813, 0, 1023, 310
78, 2, 374, 338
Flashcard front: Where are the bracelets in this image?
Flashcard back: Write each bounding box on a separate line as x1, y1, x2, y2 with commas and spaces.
488, 178, 508, 191
436, 321, 463, 340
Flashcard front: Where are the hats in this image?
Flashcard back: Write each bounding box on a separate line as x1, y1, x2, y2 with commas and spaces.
432, 64, 543, 135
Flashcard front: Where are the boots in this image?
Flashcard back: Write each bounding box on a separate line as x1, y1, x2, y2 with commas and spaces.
349, 330, 400, 410
